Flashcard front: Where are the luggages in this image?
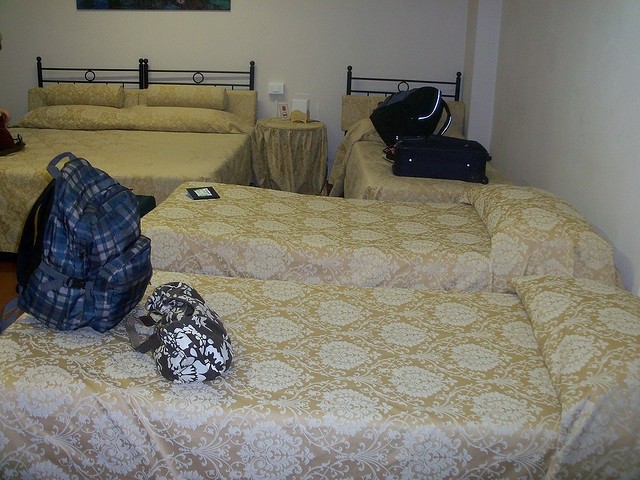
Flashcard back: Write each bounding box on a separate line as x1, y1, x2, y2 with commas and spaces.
392, 134, 492, 184
135, 194, 156, 217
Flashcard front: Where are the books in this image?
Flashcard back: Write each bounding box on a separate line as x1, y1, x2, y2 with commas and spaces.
185, 187, 220, 200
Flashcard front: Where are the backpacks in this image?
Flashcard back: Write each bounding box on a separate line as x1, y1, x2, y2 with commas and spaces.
0, 151, 152, 333
370, 86, 452, 146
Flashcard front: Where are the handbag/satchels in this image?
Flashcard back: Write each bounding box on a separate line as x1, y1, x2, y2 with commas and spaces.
126, 282, 231, 384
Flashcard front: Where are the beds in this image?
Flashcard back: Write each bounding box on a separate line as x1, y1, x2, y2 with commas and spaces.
0, 271, 639, 480
340, 65, 511, 202
0, 57, 255, 256
137, 181, 619, 291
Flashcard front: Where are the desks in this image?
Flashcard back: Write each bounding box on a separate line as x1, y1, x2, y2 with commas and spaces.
258, 118, 327, 196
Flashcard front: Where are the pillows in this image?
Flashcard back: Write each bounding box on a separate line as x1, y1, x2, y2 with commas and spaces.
25, 85, 138, 113
341, 94, 464, 140
146, 84, 228, 111
139, 87, 256, 129
45, 83, 124, 108
